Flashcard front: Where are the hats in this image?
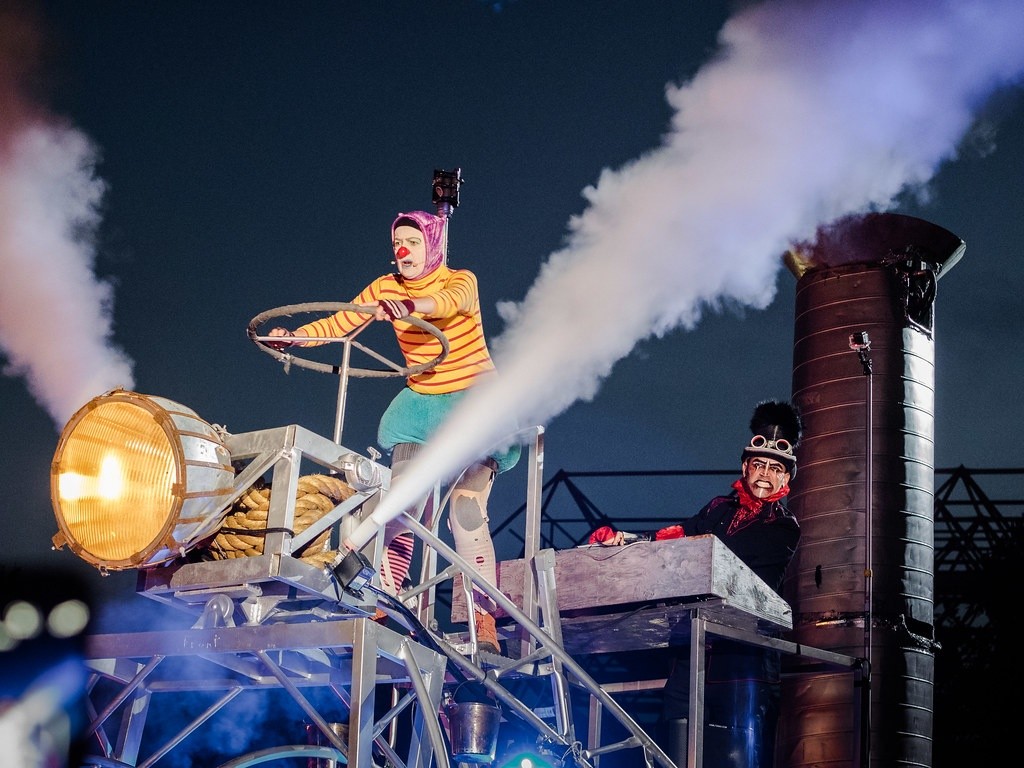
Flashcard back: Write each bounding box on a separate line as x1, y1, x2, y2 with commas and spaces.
741, 401, 803, 481
391, 211, 447, 280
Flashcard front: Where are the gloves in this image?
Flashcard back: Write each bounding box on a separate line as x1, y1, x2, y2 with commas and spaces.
589, 525, 625, 547
656, 525, 684, 542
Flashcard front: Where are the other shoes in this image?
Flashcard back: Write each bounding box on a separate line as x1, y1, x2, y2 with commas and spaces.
476, 610, 499, 655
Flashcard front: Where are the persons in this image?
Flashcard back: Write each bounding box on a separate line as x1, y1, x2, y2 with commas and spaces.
265, 211, 523, 653
589, 400, 804, 768
